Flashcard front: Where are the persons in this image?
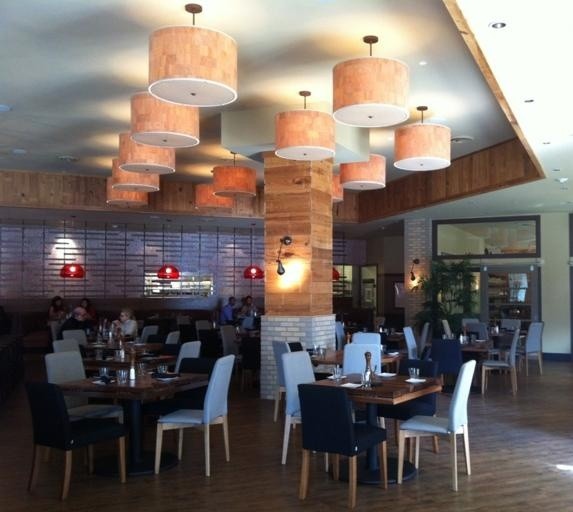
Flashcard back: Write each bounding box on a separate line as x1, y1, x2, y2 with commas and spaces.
240, 296, 256, 314
48, 295, 65, 320
220, 297, 235, 324
55, 307, 93, 340
78, 298, 96, 322
110, 307, 138, 339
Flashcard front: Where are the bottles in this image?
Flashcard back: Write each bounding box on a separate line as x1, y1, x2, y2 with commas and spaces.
362, 325, 395, 335
87, 325, 154, 359
443, 322, 500, 344
362, 351, 376, 391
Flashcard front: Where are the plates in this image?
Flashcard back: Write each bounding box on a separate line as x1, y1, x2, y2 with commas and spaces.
327, 375, 346, 379
378, 372, 396, 377
403, 379, 426, 383
340, 383, 361, 389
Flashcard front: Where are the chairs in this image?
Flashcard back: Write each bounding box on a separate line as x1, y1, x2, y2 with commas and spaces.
23, 313, 261, 503
431, 319, 544, 397
272, 315, 476, 509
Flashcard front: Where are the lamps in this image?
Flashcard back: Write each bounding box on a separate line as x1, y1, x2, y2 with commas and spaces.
60, 262, 85, 280
119, 133, 175, 174
274, 91, 336, 160
274, 234, 293, 278
393, 106, 451, 171
147, 3, 238, 107
112, 158, 160, 192
332, 266, 340, 281
332, 173, 343, 203
408, 258, 419, 282
195, 171, 234, 209
106, 176, 148, 207
333, 36, 410, 128
213, 151, 256, 197
156, 264, 180, 280
130, 91, 199, 148
243, 265, 265, 280
340, 153, 386, 190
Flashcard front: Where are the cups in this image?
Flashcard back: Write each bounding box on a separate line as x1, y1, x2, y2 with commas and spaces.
360, 369, 372, 392
408, 368, 419, 378
331, 368, 343, 380
91, 361, 177, 383
312, 342, 325, 359
